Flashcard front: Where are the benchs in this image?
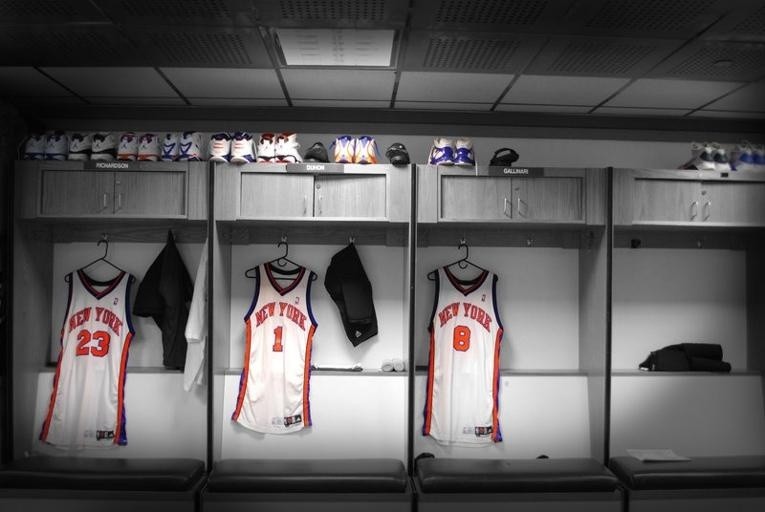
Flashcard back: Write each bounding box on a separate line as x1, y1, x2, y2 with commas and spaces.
609, 455, 765, 512
201, 459, 415, 512
0, 455, 206, 511
413, 457, 624, 512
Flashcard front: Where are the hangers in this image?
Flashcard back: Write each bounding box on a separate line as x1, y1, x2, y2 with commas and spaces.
427, 242, 498, 284
64, 239, 136, 283
245, 241, 318, 281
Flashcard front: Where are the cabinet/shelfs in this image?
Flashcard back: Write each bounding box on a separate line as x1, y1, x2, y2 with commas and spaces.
414, 165, 609, 469
213, 162, 413, 476
13, 159, 213, 472
608, 168, 765, 467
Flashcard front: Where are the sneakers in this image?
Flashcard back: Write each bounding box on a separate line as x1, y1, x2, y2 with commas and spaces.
89, 131, 118, 161
178, 129, 210, 162
453, 137, 476, 167
274, 131, 304, 165
385, 142, 411, 165
229, 130, 257, 163
67, 132, 93, 161
160, 133, 180, 162
303, 141, 331, 163
677, 142, 716, 170
710, 141, 732, 171
329, 135, 356, 163
354, 135, 379, 164
116, 130, 140, 161
255, 131, 278, 164
136, 133, 162, 162
731, 142, 754, 171
748, 142, 765, 171
206, 132, 233, 163
43, 129, 70, 161
23, 132, 50, 161
427, 136, 455, 166
